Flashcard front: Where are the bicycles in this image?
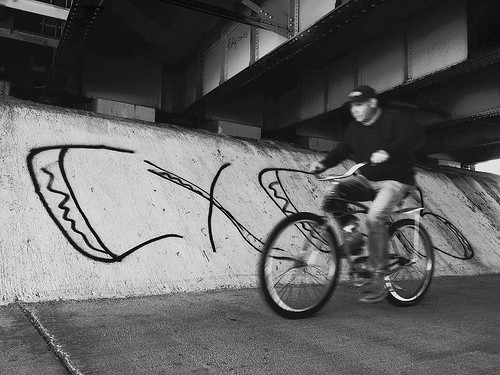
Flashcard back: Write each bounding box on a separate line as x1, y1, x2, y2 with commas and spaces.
258, 160, 435, 320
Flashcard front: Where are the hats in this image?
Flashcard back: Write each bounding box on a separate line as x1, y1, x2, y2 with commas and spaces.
342, 84, 379, 106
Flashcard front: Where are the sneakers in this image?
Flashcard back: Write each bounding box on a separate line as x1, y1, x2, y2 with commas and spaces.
358, 281, 389, 303
335, 230, 367, 257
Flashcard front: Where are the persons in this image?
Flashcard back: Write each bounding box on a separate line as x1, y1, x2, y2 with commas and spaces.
311, 86, 420, 303
